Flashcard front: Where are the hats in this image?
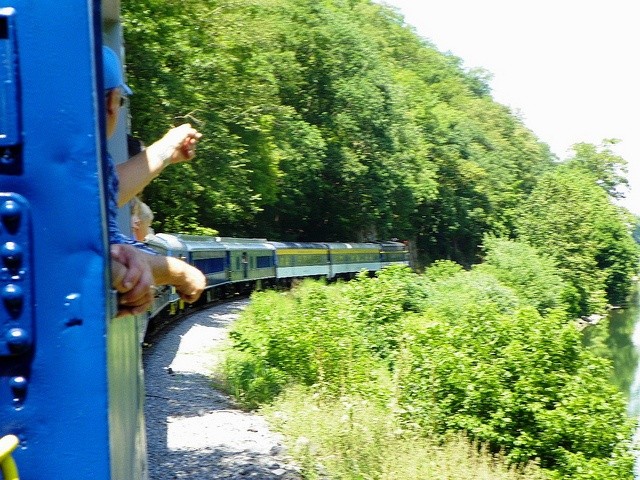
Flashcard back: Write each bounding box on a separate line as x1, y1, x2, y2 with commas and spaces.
101, 44, 131, 95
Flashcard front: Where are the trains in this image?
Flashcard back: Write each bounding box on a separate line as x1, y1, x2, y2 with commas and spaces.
0, 0, 408, 479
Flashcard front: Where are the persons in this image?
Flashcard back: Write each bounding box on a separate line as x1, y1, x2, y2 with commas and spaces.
127, 134, 142, 159
135, 202, 154, 241
101, 47, 207, 304
109, 244, 152, 318
130, 197, 139, 232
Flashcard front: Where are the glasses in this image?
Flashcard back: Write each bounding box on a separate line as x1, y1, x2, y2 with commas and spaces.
118, 94, 127, 106
